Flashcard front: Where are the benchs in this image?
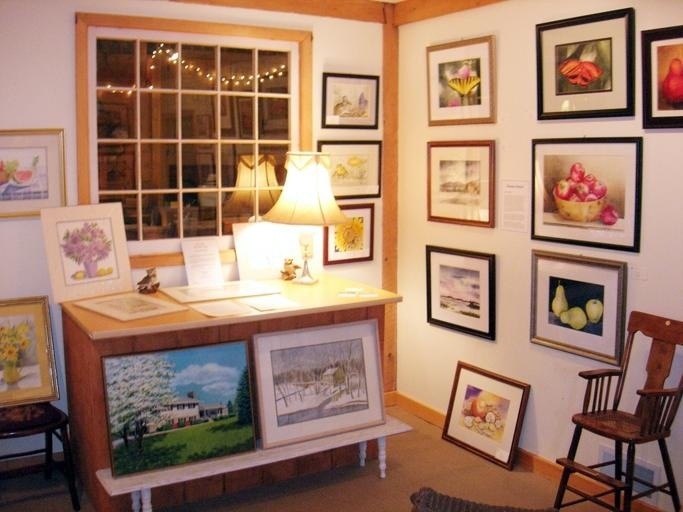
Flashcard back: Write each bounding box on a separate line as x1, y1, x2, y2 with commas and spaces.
96, 417, 414, 512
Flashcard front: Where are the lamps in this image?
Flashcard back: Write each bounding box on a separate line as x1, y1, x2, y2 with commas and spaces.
262, 152, 346, 286
223, 154, 281, 215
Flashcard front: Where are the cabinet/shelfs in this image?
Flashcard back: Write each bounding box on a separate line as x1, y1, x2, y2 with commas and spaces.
61, 274, 404, 511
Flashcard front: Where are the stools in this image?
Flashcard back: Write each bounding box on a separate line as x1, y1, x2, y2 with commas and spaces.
0, 403, 81, 510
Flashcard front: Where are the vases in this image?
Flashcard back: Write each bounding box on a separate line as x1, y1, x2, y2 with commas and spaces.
83, 261, 98, 278
4, 363, 23, 383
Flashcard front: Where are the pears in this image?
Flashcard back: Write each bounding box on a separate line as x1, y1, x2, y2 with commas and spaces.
553, 280, 603, 329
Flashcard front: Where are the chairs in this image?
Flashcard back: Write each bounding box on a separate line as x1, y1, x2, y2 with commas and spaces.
554, 311, 683, 511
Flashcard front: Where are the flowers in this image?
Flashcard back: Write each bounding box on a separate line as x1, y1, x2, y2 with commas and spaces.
61, 222, 112, 263
335, 219, 364, 252
0, 320, 30, 362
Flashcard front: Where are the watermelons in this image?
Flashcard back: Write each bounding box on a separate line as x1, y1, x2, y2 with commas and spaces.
14, 170, 33, 184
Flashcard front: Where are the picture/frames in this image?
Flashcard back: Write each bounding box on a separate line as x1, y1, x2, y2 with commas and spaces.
41, 202, 134, 305
0, 297, 59, 407
640, 26, 683, 128
323, 204, 374, 265
425, 34, 498, 127
317, 140, 382, 199
0, 128, 68, 219
535, 7, 636, 122
530, 136, 643, 253
253, 319, 386, 449
442, 361, 530, 470
529, 249, 627, 368
100, 340, 256, 476
426, 139, 495, 229
426, 245, 496, 341
321, 72, 380, 129
98, 72, 288, 196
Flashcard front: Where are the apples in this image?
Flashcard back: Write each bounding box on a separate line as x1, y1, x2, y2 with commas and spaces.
661, 58, 683, 103
555, 162, 607, 202
601, 206, 620, 224
